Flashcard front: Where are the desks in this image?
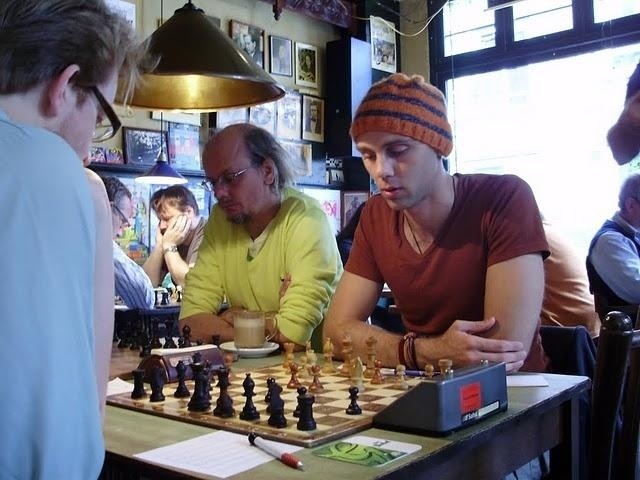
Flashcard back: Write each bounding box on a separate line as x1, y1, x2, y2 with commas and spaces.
96, 355, 593, 479
113, 290, 231, 326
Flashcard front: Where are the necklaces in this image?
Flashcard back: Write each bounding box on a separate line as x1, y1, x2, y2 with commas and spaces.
404, 176, 455, 254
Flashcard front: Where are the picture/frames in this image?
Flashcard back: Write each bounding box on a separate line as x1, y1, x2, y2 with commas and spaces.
120, 124, 171, 169
206, 13, 329, 145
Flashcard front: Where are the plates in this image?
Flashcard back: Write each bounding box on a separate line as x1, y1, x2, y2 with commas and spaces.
220, 339, 280, 359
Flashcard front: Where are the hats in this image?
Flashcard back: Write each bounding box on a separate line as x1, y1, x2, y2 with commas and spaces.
353, 73, 451, 156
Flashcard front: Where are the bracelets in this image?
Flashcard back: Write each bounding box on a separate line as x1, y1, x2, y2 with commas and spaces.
404, 333, 417, 370
163, 246, 178, 255
398, 338, 405, 365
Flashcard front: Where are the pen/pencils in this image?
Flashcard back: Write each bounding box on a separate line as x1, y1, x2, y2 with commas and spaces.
247, 433, 303, 469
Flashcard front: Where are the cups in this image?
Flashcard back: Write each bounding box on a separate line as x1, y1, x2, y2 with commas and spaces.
233, 310, 277, 349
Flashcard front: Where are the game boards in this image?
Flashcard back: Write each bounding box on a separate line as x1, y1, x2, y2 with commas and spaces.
107, 361, 425, 448
108, 334, 238, 381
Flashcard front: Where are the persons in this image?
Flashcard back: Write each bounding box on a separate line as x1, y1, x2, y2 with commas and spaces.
335, 201, 366, 267
299, 55, 315, 82
240, 33, 263, 67
273, 45, 290, 74
141, 184, 208, 288
322, 72, 550, 480
178, 122, 372, 353
305, 103, 321, 134
0, 0, 163, 480
584, 173, 640, 480
606, 63, 640, 165
102, 175, 156, 310
540, 212, 602, 340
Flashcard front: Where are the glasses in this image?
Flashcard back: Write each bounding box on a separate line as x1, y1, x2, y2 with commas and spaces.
202, 168, 248, 192
113, 205, 130, 229
92, 85, 120, 142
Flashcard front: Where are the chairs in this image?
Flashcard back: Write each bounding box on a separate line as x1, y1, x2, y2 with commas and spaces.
536, 310, 640, 480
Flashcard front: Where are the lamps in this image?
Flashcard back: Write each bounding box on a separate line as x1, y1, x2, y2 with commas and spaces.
111, 2, 287, 113
